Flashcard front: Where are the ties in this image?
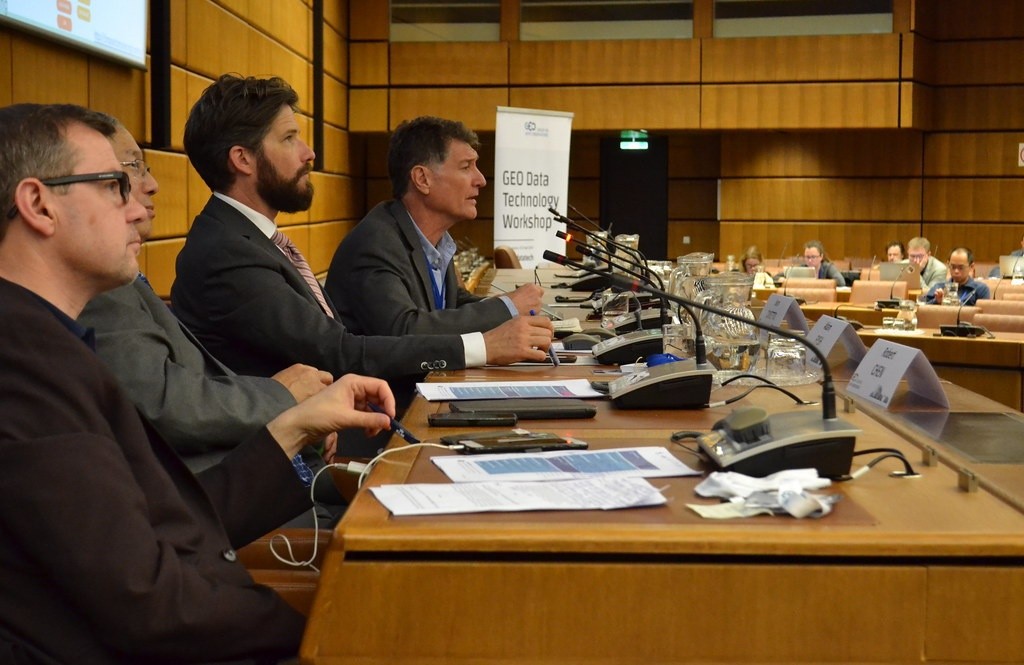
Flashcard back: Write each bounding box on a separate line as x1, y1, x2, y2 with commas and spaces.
270, 229, 334, 319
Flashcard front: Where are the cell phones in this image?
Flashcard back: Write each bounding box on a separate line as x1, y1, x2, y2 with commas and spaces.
540, 354, 577, 363
426, 412, 518, 426
458, 436, 588, 456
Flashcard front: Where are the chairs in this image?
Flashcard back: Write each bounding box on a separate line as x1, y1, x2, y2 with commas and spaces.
744, 249, 1024, 331
234, 456, 371, 615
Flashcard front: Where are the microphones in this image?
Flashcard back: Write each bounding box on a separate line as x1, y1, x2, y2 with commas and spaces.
544, 203, 1024, 480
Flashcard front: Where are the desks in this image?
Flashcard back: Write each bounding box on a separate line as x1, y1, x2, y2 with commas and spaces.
298, 262, 1024, 665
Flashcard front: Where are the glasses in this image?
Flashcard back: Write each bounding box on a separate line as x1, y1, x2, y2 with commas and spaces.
7, 170, 132, 220
119, 159, 151, 176
805, 255, 820, 259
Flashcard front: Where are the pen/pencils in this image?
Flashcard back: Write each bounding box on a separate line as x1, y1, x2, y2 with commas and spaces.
367, 400, 420, 445
530, 309, 560, 366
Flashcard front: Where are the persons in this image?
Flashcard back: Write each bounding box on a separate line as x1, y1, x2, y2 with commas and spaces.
729, 251, 776, 296
873, 235, 947, 293
989, 235, 1024, 278
76, 110, 349, 531
169, 73, 555, 458
772, 240, 846, 288
323, 115, 544, 408
925, 246, 991, 306
0, 101, 397, 665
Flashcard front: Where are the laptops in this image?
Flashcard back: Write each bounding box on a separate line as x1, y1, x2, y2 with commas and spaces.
998, 255, 1024, 278
878, 260, 923, 290
783, 266, 816, 278
